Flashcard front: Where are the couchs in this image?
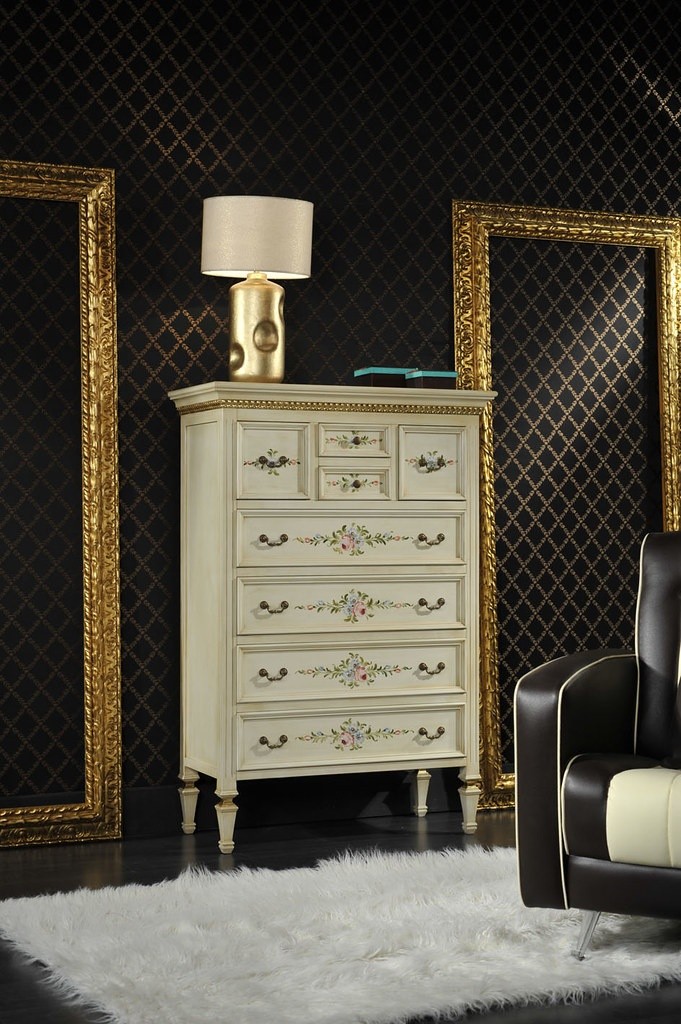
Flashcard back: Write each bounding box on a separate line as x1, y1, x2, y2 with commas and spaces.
514, 532, 681, 961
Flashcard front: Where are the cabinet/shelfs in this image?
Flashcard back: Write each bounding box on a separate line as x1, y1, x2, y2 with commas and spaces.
167, 381, 500, 856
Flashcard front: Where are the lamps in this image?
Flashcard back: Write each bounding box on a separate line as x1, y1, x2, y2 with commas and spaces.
200, 196, 314, 384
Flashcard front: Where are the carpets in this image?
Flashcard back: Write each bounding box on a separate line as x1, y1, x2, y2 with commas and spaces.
0, 843, 681, 1024
